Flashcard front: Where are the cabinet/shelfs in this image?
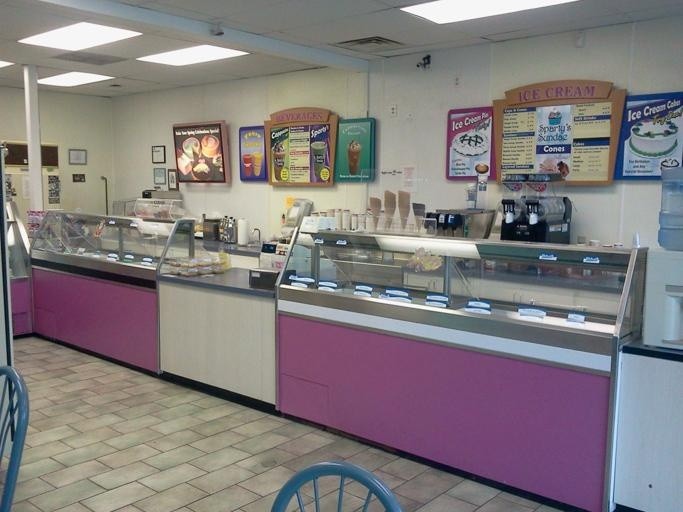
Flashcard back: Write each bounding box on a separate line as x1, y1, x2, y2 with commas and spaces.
4, 276, 34, 337
158, 274, 278, 411
609, 338, 683, 512
30, 208, 195, 375
274, 224, 648, 512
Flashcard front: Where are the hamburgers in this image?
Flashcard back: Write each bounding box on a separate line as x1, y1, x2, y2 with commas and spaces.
537, 157, 561, 174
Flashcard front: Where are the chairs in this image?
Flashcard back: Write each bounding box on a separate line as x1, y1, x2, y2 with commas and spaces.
268, 463, 402, 512
0, 364, 31, 511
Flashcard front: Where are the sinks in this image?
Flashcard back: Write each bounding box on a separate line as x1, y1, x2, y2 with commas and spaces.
247, 243, 262, 250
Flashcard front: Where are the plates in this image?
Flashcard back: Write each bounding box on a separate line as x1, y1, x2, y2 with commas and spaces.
475, 164, 489, 173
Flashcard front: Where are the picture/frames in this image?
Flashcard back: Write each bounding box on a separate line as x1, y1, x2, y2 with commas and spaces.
153, 167, 166, 185
151, 145, 166, 164
68, 149, 87, 165
168, 168, 179, 190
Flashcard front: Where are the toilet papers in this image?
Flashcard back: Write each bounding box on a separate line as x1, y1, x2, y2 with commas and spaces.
237, 217, 250, 247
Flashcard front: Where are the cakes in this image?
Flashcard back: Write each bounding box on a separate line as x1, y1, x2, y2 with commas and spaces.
182, 137, 200, 160
452, 131, 489, 155
627, 118, 679, 158
191, 158, 211, 181
550, 112, 561, 124
200, 135, 219, 158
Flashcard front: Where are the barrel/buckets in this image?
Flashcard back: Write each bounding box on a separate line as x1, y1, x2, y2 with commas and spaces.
657, 167, 683, 251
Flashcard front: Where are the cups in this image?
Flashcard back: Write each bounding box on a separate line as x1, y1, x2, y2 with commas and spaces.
273, 148, 285, 180
589, 240, 600, 246
253, 152, 263, 176
348, 143, 362, 176
548, 117, 563, 124
311, 142, 327, 177
243, 154, 252, 177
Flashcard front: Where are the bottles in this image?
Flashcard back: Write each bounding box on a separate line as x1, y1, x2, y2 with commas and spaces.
311, 211, 319, 216
365, 208, 376, 231
335, 208, 343, 231
342, 209, 352, 231
219, 216, 237, 244
351, 213, 358, 232
326, 208, 335, 231
319, 210, 328, 218
377, 209, 386, 231
356, 214, 367, 231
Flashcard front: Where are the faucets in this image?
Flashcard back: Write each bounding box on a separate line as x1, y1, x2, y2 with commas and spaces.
250, 227, 261, 242
